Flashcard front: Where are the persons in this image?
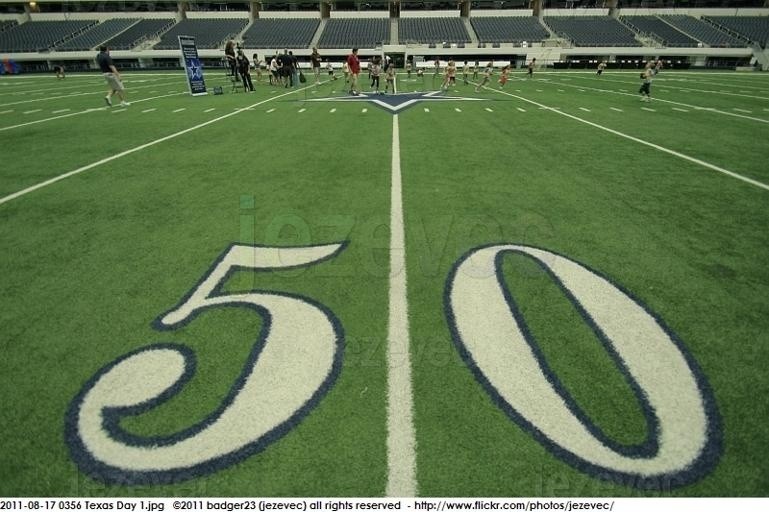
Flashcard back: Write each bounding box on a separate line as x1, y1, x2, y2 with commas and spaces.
222, 40, 511, 96
525, 57, 536, 77
51, 63, 66, 80
655, 57, 665, 74
96, 44, 130, 108
595, 60, 608, 76
639, 62, 655, 103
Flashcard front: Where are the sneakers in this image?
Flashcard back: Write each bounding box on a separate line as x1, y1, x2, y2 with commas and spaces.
105, 95, 131, 110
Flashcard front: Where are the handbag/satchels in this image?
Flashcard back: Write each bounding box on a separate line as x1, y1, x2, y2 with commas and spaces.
299, 72, 307, 83
